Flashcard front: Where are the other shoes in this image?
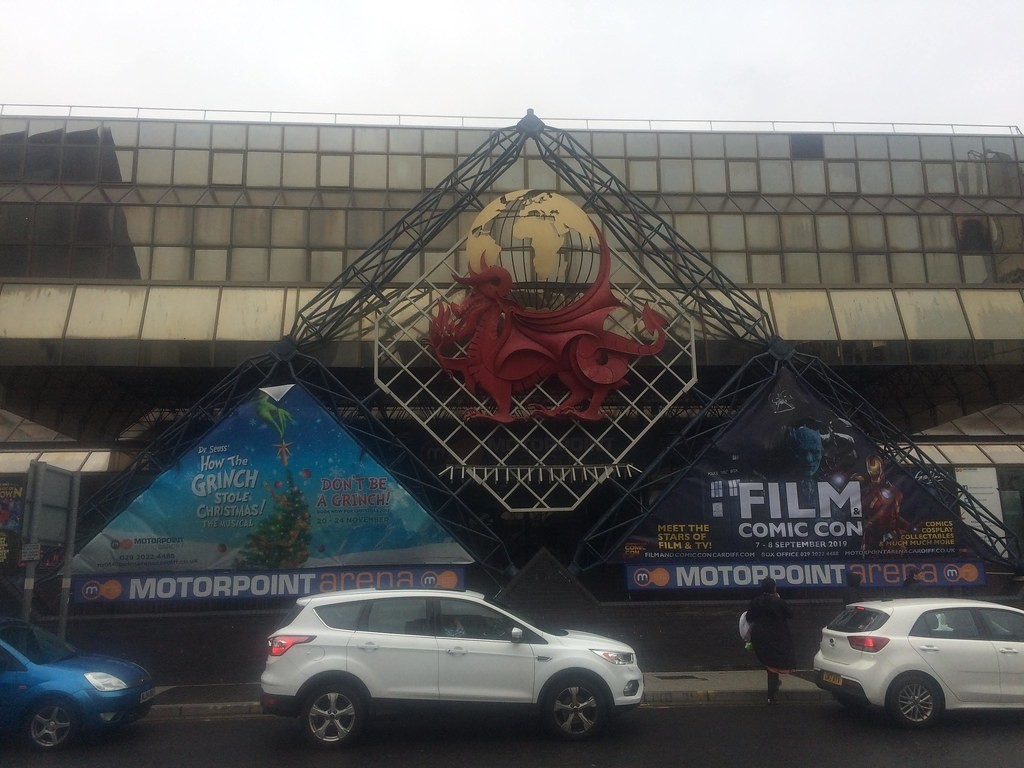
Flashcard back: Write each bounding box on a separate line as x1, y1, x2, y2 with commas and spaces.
767, 698, 775, 705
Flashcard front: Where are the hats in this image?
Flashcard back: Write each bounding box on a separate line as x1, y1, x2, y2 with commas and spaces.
761, 575, 776, 592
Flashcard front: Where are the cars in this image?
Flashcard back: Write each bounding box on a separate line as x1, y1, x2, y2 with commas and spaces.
0, 617, 156, 753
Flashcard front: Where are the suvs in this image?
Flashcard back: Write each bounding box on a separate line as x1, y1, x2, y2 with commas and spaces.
258, 586, 645, 751
812, 596, 1024, 732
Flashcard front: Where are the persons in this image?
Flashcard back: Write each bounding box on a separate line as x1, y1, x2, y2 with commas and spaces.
781, 426, 845, 521
850, 453, 920, 561
440, 606, 466, 638
745, 576, 797, 705
844, 569, 929, 605
